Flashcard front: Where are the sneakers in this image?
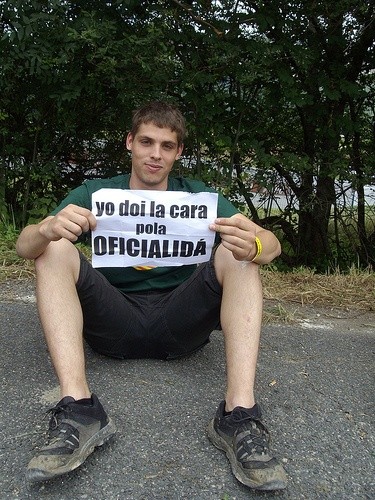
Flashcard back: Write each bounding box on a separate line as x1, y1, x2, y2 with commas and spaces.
207, 399, 287, 491
26, 393, 116, 482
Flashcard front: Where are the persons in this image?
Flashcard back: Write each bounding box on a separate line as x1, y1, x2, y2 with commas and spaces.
15, 101, 289, 491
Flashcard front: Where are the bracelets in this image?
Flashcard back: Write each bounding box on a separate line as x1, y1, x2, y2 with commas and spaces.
250, 236, 263, 261
